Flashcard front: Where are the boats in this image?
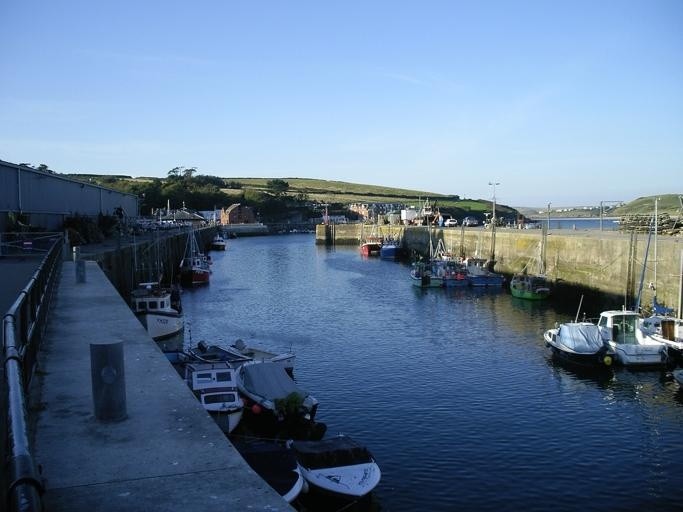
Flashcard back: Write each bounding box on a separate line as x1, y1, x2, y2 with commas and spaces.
358, 235, 403, 258
213, 234, 225, 250
162, 339, 382, 503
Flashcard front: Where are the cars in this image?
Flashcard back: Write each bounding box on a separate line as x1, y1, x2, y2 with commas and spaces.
136, 218, 192, 230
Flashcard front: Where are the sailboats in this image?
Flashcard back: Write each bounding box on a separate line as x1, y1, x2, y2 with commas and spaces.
179, 224, 213, 286
130, 228, 184, 339
407, 201, 505, 289
509, 223, 551, 300
543, 199, 683, 366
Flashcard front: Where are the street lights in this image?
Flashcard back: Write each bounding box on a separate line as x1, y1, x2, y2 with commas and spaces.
488, 182, 496, 269
